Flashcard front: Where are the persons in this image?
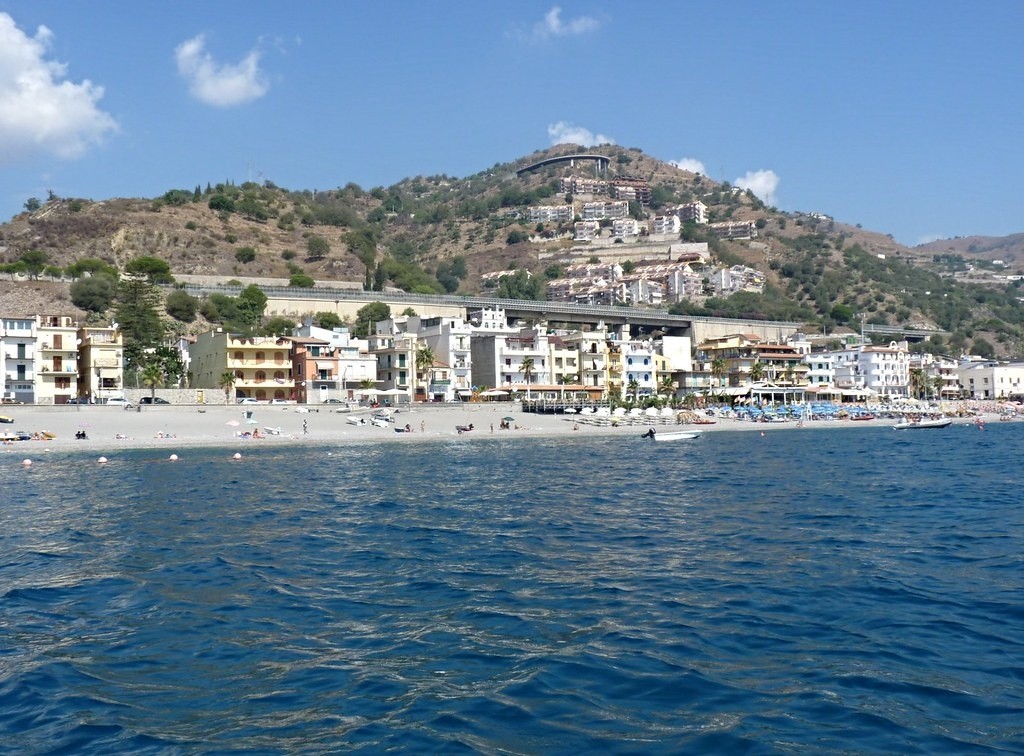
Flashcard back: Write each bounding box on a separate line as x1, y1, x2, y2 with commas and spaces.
75, 431, 86, 439
879, 401, 1024, 428
421, 420, 425, 433
157, 431, 176, 439
361, 419, 365, 424
303, 419, 309, 434
253, 428, 258, 438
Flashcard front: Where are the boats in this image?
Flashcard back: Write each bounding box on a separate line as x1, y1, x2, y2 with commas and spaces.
894, 419, 951, 429
653, 429, 704, 441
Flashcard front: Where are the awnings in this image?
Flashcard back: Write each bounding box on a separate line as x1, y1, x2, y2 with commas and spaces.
458, 391, 472, 396
706, 389, 750, 396
942, 392, 960, 395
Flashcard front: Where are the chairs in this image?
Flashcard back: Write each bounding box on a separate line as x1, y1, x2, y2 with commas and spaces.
265, 427, 284, 435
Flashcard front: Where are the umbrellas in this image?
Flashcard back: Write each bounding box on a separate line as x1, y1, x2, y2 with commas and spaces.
564, 406, 686, 418
706, 400, 879, 418
502, 417, 514, 423
356, 388, 408, 403
479, 391, 509, 402
